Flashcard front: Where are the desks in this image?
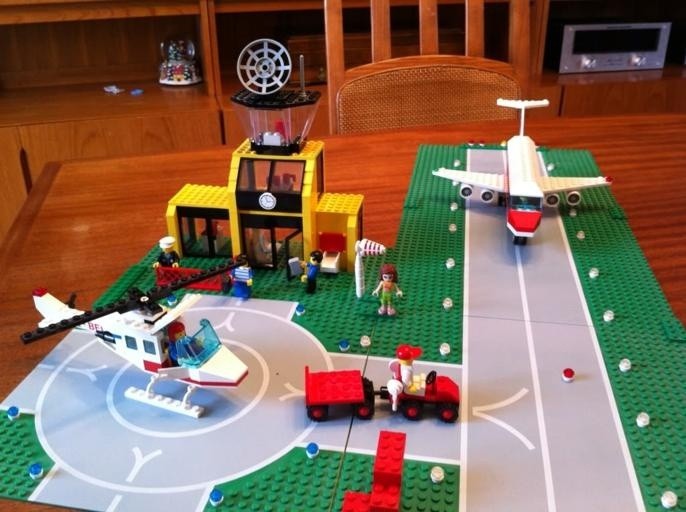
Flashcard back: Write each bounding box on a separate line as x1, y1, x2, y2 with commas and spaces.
0, 112, 686, 512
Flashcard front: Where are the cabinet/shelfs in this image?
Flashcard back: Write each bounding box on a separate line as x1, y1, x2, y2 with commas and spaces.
0, 82, 226, 237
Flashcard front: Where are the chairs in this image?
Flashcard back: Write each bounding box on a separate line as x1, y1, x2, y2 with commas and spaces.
324, 0, 532, 135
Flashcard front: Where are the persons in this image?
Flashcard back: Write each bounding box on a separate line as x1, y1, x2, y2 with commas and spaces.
153, 41, 402, 315
